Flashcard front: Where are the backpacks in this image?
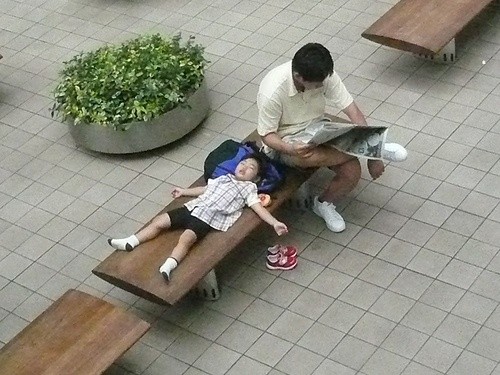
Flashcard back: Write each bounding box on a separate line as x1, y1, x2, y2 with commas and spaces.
204, 139, 285, 193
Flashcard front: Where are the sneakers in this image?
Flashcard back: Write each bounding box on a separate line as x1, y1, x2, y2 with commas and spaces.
383, 142, 408, 162
312, 194, 346, 233
266, 244, 298, 271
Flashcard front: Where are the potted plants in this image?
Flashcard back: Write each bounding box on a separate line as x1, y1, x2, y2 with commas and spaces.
48, 31, 213, 154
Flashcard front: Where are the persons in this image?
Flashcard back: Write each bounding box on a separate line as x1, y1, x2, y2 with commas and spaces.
256, 42, 409, 233
108, 153, 288, 280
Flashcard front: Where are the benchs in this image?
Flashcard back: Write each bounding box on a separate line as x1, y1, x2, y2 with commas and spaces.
91, 111, 357, 306
0, 288, 152, 375
360, 0, 493, 64
